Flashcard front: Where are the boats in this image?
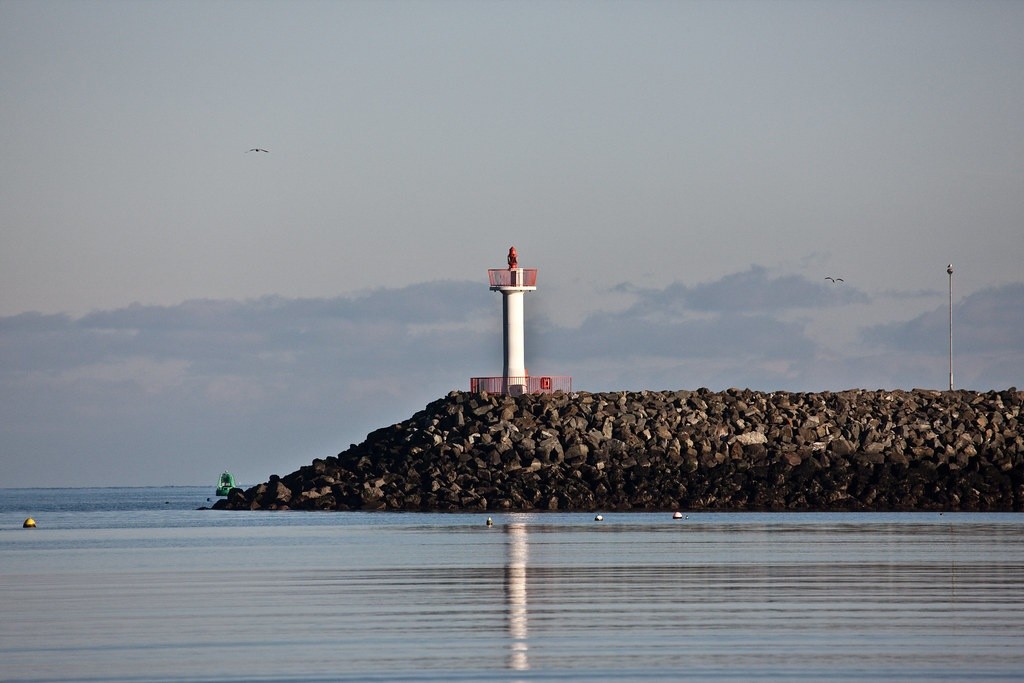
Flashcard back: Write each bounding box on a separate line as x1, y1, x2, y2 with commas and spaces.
216, 469, 236, 496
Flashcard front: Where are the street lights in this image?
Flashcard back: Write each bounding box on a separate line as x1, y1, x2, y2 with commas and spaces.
947, 269, 955, 391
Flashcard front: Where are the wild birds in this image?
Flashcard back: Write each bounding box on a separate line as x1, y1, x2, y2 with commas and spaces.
245, 148, 269, 153
824, 277, 844, 282
